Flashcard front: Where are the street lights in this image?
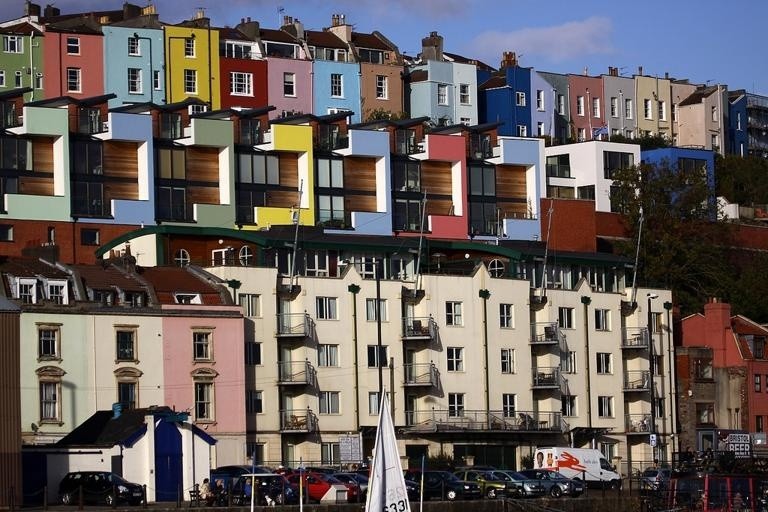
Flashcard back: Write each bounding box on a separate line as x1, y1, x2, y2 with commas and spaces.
644, 292, 660, 469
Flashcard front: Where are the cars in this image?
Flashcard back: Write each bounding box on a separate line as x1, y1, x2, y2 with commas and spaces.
56, 470, 146, 509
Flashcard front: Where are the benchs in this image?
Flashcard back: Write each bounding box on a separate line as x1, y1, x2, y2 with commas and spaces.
188, 490, 242, 507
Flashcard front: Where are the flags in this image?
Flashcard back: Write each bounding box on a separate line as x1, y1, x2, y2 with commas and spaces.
361, 386, 416, 512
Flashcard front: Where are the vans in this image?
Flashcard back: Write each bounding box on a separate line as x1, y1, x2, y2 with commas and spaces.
531, 446, 621, 490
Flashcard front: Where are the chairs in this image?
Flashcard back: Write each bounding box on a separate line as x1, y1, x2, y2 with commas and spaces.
406, 320, 428, 336
288, 415, 300, 430
542, 326, 555, 342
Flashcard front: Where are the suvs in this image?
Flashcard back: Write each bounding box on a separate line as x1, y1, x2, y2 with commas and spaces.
640, 468, 676, 490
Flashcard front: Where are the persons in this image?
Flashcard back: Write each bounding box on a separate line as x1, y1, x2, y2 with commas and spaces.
537, 452, 544, 467
244, 478, 252, 498
254, 479, 261, 506
211, 480, 229, 507
199, 478, 215, 506
